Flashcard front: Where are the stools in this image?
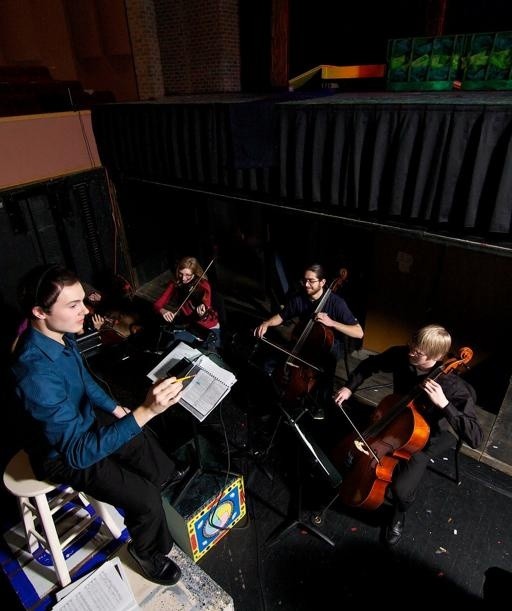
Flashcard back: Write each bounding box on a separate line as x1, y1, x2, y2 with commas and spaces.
3, 449, 122, 589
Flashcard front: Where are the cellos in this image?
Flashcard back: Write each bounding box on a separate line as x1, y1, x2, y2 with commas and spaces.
277, 268, 347, 404
338, 347, 473, 511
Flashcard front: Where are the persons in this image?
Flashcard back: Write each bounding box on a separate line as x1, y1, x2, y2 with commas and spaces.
12, 275, 184, 586
91, 314, 136, 360
253, 264, 364, 420
154, 258, 221, 351
334, 324, 484, 547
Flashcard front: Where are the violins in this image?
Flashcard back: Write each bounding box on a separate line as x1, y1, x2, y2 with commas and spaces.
171, 278, 218, 320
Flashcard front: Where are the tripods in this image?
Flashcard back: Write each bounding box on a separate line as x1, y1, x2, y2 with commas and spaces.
266, 472, 343, 548
170, 410, 240, 511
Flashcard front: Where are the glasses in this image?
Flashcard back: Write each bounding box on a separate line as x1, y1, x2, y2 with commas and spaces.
407, 341, 427, 357
300, 279, 320, 286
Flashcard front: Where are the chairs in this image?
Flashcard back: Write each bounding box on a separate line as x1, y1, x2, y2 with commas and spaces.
431, 375, 478, 486
328, 326, 352, 408
198, 291, 226, 355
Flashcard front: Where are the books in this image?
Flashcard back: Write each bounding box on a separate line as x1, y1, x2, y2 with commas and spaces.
148, 341, 236, 422
50, 556, 141, 611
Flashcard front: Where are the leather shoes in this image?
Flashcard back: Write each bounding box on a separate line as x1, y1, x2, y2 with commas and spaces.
385, 511, 407, 547
125, 539, 182, 586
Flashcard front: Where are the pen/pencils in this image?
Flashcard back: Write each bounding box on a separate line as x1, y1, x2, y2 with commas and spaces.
174, 372, 197, 384
